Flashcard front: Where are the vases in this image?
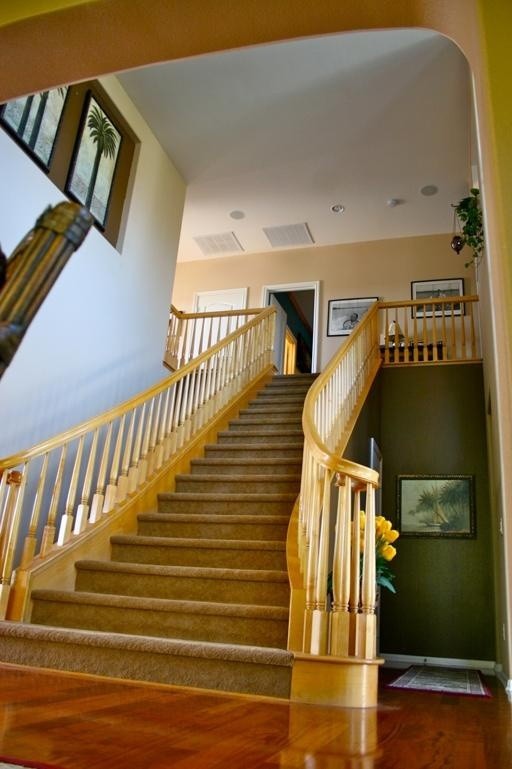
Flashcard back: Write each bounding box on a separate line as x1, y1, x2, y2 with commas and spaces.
329, 588, 379, 614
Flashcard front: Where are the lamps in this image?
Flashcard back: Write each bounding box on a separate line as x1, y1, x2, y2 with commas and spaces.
384, 320, 405, 344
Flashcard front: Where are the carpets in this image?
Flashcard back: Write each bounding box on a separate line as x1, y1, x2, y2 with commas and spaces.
384, 665, 494, 700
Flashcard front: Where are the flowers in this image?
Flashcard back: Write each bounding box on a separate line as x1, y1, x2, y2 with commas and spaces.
326, 511, 401, 596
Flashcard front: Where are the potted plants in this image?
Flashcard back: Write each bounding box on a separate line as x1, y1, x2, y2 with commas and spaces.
453, 189, 486, 269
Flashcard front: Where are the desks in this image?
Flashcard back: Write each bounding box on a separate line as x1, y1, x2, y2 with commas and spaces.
380, 341, 443, 362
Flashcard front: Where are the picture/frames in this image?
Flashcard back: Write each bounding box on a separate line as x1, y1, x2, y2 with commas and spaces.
411, 278, 466, 319
0, 85, 73, 175
370, 437, 382, 516
64, 89, 123, 232
327, 297, 379, 337
396, 473, 477, 540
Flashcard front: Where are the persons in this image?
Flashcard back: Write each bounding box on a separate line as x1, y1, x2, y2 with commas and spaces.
342, 312, 359, 330
